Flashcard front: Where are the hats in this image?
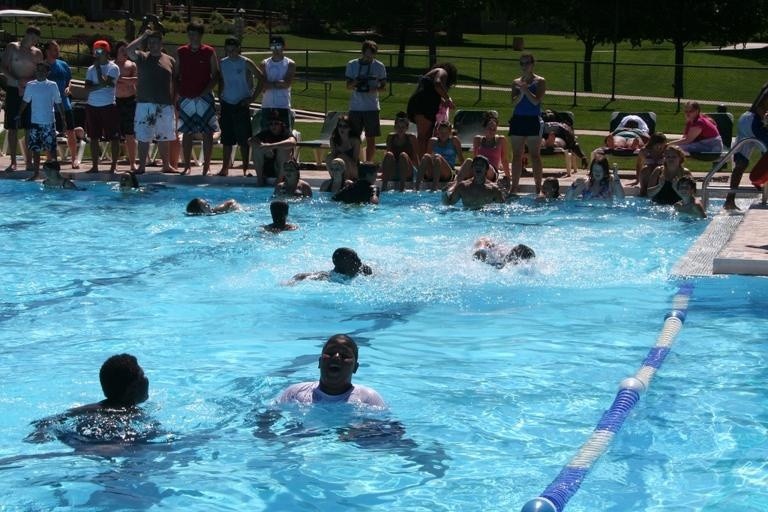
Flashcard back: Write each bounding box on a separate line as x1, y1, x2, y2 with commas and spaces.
149, 31, 164, 39
93, 40, 111, 52
225, 36, 241, 45
270, 35, 285, 46
44, 158, 60, 169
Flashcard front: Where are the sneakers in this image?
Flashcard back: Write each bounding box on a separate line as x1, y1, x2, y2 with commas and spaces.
472, 163, 485, 169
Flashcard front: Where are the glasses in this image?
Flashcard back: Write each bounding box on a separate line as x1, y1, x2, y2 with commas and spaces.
438, 124, 449, 128
284, 168, 296, 173
684, 110, 693, 113
519, 61, 534, 66
269, 122, 282, 126
42, 164, 52, 169
330, 168, 339, 171
395, 125, 406, 129
338, 124, 349, 129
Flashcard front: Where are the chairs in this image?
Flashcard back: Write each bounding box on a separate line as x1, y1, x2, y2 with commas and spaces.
591, 111, 656, 174
524, 110, 577, 177
363, 120, 418, 163
294, 111, 349, 164
18, 108, 301, 165
454, 109, 498, 151
682, 112, 736, 175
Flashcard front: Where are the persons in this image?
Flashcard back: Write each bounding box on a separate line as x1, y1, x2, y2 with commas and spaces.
510, 55, 546, 202
174, 23, 218, 176
568, 157, 625, 207
536, 179, 561, 203
319, 159, 380, 204
418, 121, 463, 189
218, 37, 264, 175
458, 112, 510, 181
380, 111, 419, 193
1, 14, 175, 189
539, 108, 590, 168
346, 40, 387, 163
473, 238, 536, 269
248, 35, 313, 196
187, 198, 241, 215
724, 83, 767, 209
293, 247, 372, 285
667, 103, 722, 159
627, 135, 707, 219
274, 334, 387, 409
607, 117, 648, 152
442, 155, 506, 207
46, 354, 150, 423
407, 62, 457, 147
261, 202, 297, 232
326, 115, 363, 180
